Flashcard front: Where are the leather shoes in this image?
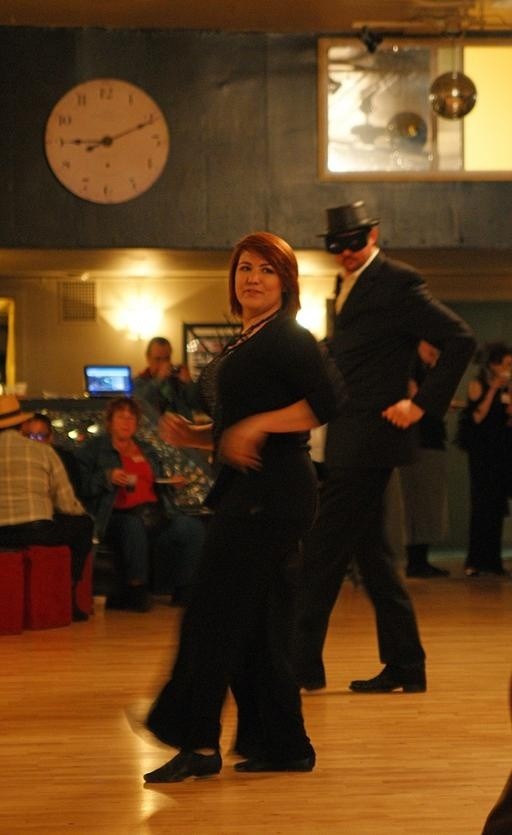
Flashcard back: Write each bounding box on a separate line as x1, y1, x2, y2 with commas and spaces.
144, 749, 221, 783
299, 670, 325, 689
348, 663, 426, 693
235, 743, 315, 771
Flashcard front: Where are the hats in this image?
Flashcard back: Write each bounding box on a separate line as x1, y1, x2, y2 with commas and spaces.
315, 200, 380, 237
0, 395, 33, 429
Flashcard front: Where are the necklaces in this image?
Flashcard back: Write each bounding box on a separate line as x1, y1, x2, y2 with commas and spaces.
229, 307, 284, 346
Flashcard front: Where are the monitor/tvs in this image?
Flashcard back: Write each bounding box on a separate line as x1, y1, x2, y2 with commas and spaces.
84, 365, 133, 394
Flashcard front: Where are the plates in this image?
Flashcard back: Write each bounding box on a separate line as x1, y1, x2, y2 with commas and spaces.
156, 479, 182, 484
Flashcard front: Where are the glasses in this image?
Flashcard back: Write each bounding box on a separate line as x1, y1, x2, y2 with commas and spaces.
323, 237, 367, 253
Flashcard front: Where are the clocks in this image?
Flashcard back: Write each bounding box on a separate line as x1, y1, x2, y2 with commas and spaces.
41, 78, 171, 209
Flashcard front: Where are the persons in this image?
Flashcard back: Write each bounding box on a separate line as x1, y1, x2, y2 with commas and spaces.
142, 231, 350, 783
2, 337, 216, 625
397, 336, 459, 579
297, 200, 476, 694
462, 341, 512, 578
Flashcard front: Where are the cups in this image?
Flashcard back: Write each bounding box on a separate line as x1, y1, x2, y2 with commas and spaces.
125, 475, 137, 493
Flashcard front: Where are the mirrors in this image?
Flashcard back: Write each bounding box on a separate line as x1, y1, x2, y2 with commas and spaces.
315, 33, 512, 185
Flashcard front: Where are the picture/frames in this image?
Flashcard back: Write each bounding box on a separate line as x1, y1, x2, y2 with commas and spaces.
182, 321, 246, 383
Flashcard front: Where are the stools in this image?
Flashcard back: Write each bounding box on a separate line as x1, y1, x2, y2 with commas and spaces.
0, 543, 109, 638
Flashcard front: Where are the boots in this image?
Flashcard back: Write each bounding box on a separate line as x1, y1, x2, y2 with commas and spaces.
404, 547, 447, 578
